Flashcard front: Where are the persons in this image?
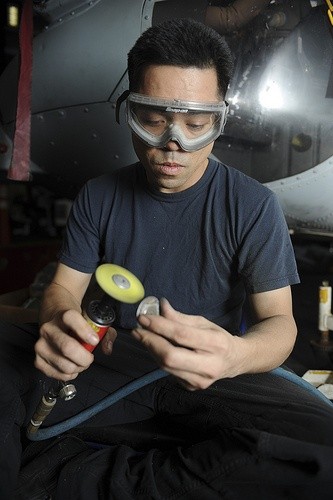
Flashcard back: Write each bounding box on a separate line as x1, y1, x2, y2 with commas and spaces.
32, 19, 303, 393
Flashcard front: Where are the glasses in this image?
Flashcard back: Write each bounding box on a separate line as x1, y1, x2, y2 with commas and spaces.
116, 91, 229, 152
